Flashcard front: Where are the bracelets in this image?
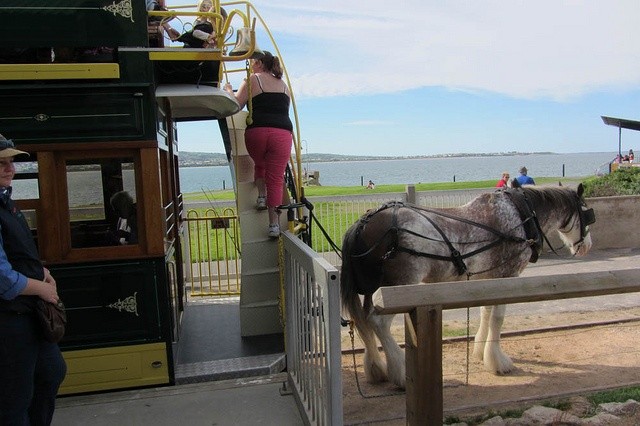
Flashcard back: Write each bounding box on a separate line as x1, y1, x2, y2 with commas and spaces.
165, 27, 173, 32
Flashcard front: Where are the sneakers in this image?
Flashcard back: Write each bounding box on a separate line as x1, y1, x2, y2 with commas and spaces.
269, 223, 280, 237
148, 4, 176, 25
257, 196, 267, 210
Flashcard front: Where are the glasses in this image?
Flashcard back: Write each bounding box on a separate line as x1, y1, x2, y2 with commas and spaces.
505, 177, 509, 178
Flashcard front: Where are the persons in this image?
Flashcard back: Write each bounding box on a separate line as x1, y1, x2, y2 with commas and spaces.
195, 0, 227, 31
616, 148, 634, 162
145, 0, 178, 49
110, 189, 139, 244
176, 6, 227, 47
517, 167, 535, 187
367, 179, 374, 189
223, 51, 293, 238
496, 172, 510, 187
0, 135, 66, 426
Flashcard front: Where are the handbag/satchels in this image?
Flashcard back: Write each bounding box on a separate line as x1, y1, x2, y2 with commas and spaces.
32, 295, 68, 341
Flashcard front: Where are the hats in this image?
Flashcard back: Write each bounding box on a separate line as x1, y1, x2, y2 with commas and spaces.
0, 134, 30, 158
520, 167, 527, 173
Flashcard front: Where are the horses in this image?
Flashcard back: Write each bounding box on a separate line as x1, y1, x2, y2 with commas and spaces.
340, 181, 592, 389
